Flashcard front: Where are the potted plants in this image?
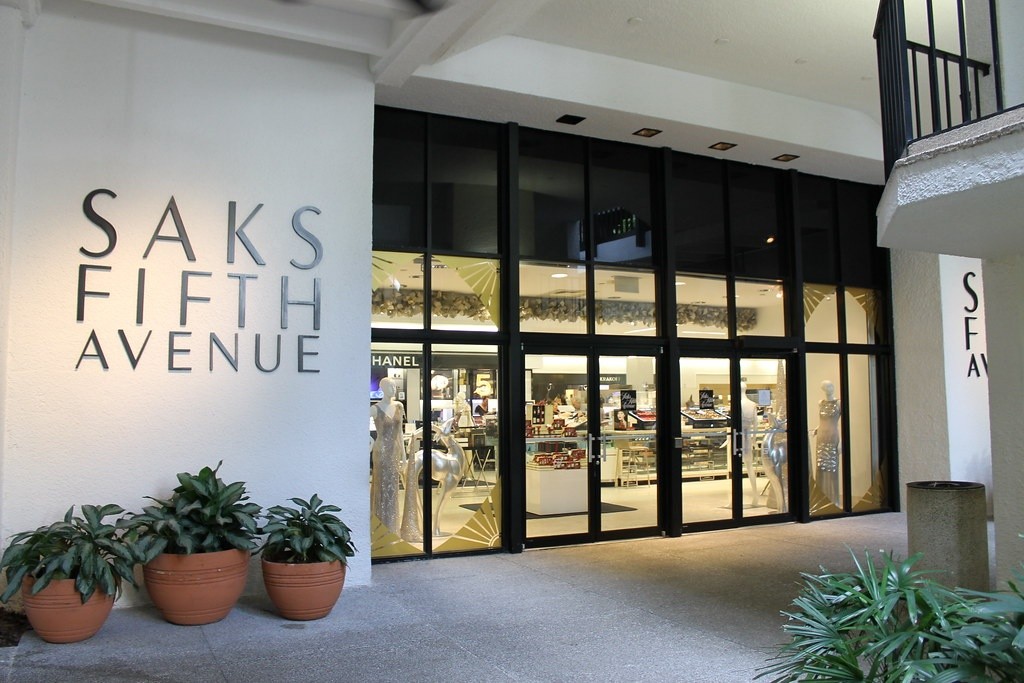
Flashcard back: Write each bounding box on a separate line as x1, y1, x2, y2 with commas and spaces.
247, 494, 360, 623
115, 458, 266, 627
0, 503, 141, 646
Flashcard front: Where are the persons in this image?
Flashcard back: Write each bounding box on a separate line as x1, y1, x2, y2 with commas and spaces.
719, 381, 758, 508
370, 378, 407, 538
475, 396, 489, 416
812, 381, 841, 507
615, 411, 627, 429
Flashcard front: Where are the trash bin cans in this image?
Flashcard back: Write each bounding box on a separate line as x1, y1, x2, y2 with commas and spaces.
904, 480, 991, 599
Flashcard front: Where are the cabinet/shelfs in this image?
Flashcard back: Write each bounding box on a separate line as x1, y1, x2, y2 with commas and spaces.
370, 398, 501, 484
618, 405, 774, 480
526, 398, 618, 514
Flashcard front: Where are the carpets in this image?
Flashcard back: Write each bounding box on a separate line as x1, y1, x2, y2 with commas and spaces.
398, 478, 495, 490
460, 501, 637, 519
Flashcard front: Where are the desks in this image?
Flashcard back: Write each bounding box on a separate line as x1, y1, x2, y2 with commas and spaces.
436, 443, 494, 490
